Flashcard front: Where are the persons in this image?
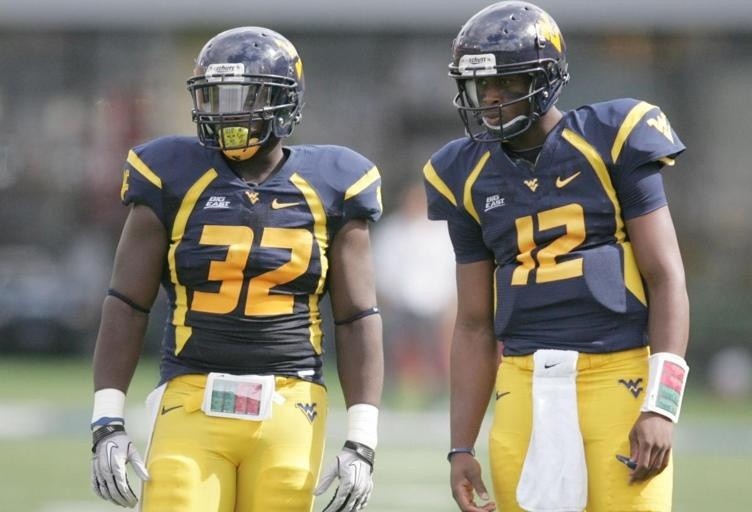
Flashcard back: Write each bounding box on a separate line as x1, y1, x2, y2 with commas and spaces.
416, 1, 691, 512
369, 180, 462, 385
88, 23, 386, 512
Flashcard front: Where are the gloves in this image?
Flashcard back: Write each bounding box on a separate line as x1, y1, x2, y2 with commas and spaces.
89, 420, 151, 509
312, 440, 376, 512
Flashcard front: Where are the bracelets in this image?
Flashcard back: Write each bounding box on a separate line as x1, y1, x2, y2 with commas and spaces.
446, 445, 476, 463
640, 351, 690, 423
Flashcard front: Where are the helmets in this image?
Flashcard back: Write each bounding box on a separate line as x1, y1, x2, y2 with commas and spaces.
194, 25, 306, 137
448, 1, 569, 116
